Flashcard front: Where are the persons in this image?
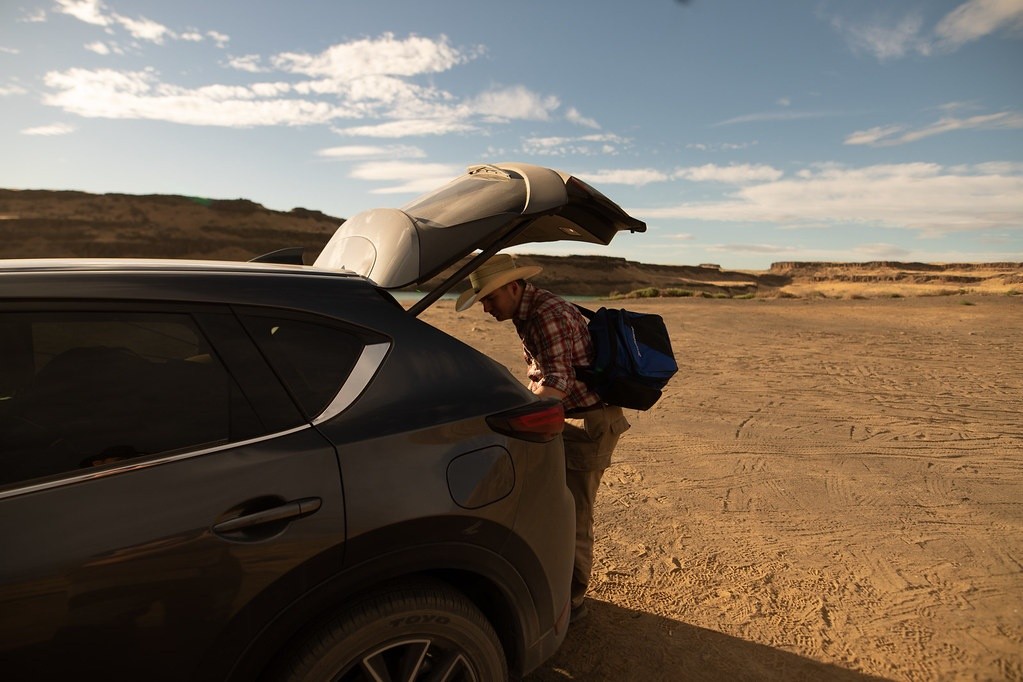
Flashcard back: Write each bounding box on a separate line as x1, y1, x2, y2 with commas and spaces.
455, 253, 631, 622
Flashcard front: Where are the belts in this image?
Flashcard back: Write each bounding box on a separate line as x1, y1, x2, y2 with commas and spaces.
566, 400, 603, 413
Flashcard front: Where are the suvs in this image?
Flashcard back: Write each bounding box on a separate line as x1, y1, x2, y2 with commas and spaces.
1, 163, 647, 682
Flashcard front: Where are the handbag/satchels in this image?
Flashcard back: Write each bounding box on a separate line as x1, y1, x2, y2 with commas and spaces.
589, 307, 678, 411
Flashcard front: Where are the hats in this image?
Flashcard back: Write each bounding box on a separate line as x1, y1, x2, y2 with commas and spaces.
455, 254, 543, 312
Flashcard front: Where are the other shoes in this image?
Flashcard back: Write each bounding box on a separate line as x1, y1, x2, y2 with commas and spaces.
569, 601, 588, 623
